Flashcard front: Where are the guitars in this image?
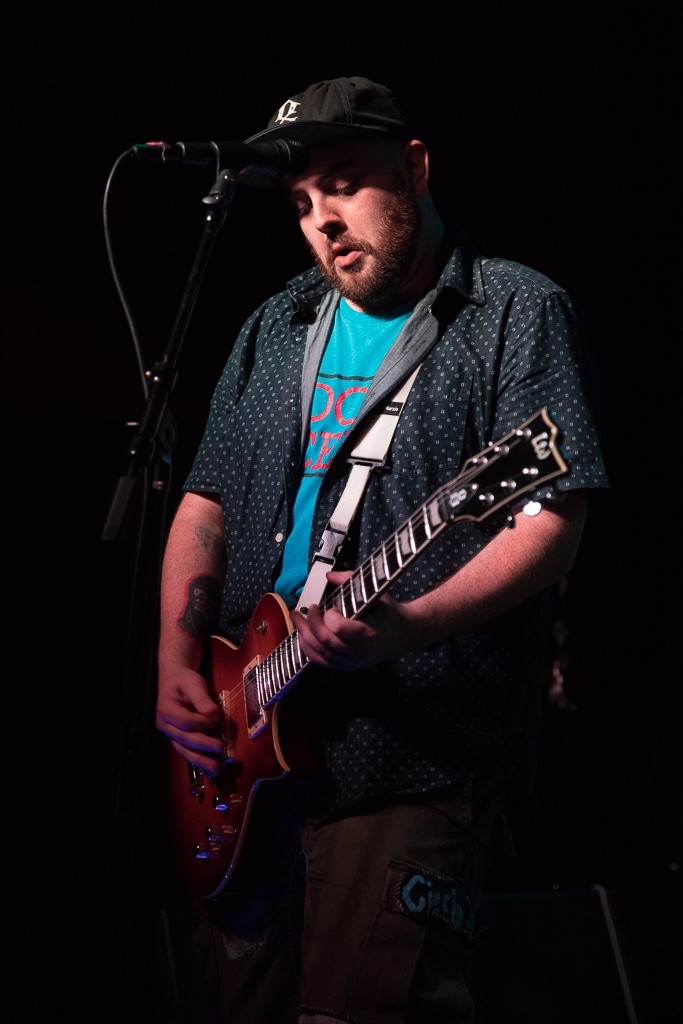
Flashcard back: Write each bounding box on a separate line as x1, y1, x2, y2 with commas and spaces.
164, 405, 572, 906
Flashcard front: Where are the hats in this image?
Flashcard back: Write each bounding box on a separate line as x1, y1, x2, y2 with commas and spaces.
244, 77, 406, 144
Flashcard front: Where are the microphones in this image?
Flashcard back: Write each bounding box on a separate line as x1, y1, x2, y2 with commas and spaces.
132, 140, 311, 172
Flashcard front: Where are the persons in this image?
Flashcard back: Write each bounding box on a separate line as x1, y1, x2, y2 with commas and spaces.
156, 76, 609, 1024
